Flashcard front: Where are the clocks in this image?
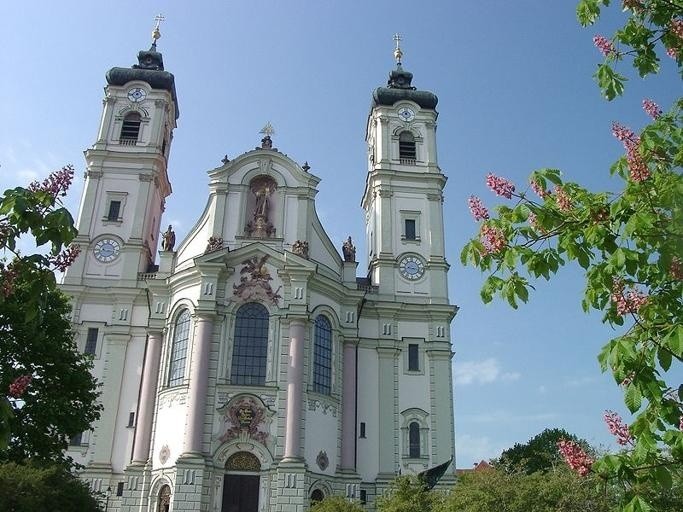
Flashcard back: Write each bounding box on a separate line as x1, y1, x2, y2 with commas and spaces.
128, 88, 146, 102
395, 251, 426, 282
398, 107, 414, 121
93, 237, 120, 265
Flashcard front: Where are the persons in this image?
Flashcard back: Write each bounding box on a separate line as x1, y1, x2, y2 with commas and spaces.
162, 225, 174, 252
256, 182, 270, 216
343, 237, 353, 261
